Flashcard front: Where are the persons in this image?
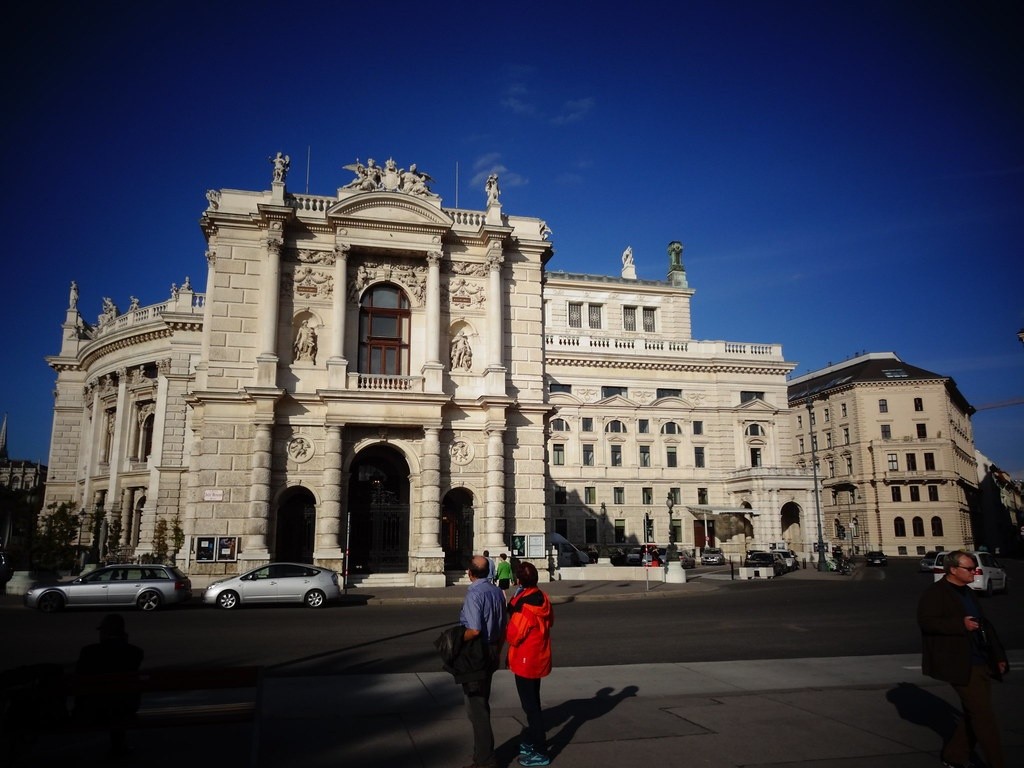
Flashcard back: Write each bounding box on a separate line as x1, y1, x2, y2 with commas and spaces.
268, 150, 290, 181
171, 275, 194, 302
341, 158, 434, 196
293, 321, 315, 362
493, 553, 514, 607
640, 545, 660, 567
483, 550, 496, 583
486, 171, 503, 200
919, 551, 1009, 768
70, 280, 78, 309
503, 561, 554, 766
451, 331, 473, 370
129, 295, 139, 309
28, 555, 63, 582
97, 296, 115, 324
441, 555, 507, 768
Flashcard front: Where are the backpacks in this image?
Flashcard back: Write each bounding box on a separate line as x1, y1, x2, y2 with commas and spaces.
964, 614, 1008, 684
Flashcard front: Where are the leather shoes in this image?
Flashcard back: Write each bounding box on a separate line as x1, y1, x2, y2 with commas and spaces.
462, 759, 498, 767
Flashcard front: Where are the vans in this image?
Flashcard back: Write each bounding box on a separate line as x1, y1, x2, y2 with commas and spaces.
553, 533, 591, 564
934, 552, 1008, 597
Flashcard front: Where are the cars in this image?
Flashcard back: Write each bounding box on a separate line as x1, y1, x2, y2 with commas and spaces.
676, 549, 695, 568
745, 549, 797, 575
700, 548, 725, 565
201, 563, 340, 608
865, 552, 887, 567
920, 550, 939, 572
827, 557, 847, 571
26, 564, 192, 613
625, 548, 642, 564
642, 547, 668, 567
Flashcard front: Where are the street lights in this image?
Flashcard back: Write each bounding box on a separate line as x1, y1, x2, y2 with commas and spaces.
666, 494, 677, 561
76, 508, 86, 572
806, 397, 830, 572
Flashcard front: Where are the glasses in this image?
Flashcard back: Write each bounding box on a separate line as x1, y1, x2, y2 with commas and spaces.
957, 565, 976, 571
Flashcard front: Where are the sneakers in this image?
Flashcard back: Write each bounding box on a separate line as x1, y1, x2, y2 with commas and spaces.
940, 750, 979, 768
517, 739, 550, 765
